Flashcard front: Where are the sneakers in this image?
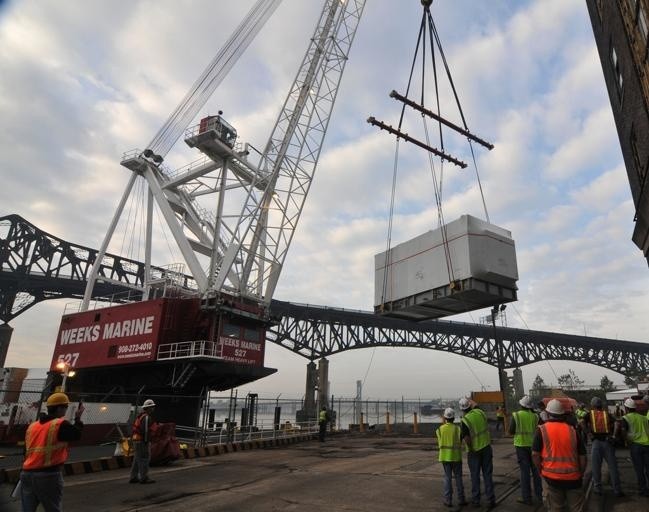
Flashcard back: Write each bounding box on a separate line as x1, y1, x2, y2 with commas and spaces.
591, 485, 625, 495
129, 478, 156, 484
444, 498, 544, 505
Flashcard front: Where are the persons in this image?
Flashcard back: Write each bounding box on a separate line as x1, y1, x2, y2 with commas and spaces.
128, 398, 161, 484
432, 394, 649, 512
18, 393, 86, 512
318, 406, 332, 443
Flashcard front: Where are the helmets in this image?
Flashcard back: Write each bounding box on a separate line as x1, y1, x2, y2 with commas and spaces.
458, 399, 470, 411
443, 407, 455, 419
46, 393, 70, 407
545, 399, 565, 415
518, 395, 534, 409
143, 400, 158, 408
624, 399, 637, 409
591, 397, 602, 408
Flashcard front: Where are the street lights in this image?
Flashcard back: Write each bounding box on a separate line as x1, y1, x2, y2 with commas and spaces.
489, 303, 507, 413
54, 360, 77, 395
